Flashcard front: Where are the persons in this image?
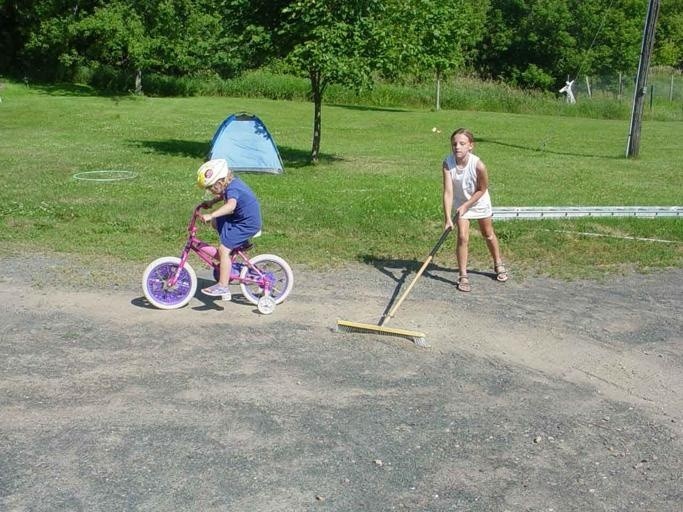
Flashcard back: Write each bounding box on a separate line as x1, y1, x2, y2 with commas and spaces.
558, 80, 577, 103
196, 157, 262, 296
443, 129, 507, 292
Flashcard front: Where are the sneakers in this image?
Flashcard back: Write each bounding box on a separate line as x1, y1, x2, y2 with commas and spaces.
201, 282, 231, 296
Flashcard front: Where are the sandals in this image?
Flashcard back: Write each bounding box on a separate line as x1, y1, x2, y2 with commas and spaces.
457, 275, 471, 292
494, 262, 508, 282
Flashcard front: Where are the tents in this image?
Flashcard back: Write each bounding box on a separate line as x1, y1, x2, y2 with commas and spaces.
207, 112, 287, 175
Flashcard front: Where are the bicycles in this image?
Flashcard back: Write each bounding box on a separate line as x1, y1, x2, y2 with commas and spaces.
140, 203, 291, 314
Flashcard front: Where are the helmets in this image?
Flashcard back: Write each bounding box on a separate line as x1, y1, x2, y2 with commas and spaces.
197, 158, 228, 190
